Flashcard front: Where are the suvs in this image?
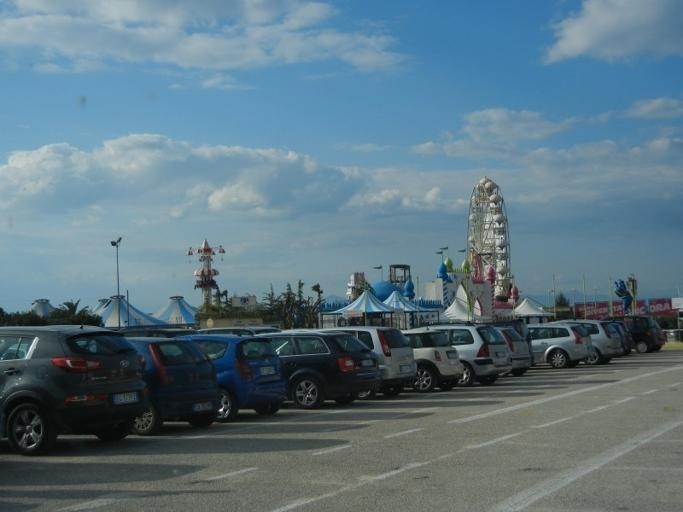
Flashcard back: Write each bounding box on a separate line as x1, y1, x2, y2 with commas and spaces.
522, 322, 594, 369
174, 332, 288, 423
539, 315, 668, 366
75, 336, 220, 437
116, 325, 283, 338
276, 326, 418, 400
0, 324, 151, 457
421, 325, 515, 388
398, 328, 464, 393
208, 328, 383, 410
487, 326, 532, 377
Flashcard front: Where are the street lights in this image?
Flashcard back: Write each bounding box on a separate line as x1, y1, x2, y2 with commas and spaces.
111, 237, 122, 327
